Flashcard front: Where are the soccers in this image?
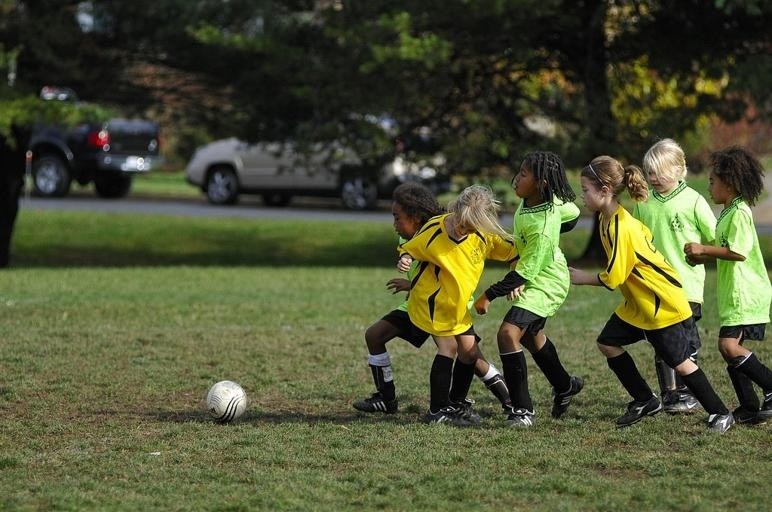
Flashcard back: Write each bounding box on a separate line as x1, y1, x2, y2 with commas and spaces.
206, 379, 246, 422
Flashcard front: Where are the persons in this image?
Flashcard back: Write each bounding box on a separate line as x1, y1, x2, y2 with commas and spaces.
567, 155, 739, 431
632, 136, 718, 414
395, 183, 525, 428
474, 148, 583, 425
350, 180, 519, 420
682, 144, 771, 425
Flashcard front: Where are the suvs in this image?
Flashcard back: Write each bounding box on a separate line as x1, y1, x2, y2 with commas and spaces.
26, 88, 156, 198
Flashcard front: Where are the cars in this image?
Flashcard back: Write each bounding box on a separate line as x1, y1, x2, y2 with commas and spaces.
184, 114, 450, 209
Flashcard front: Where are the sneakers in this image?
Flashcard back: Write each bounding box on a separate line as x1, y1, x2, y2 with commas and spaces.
759, 392, 772, 414
503, 407, 536, 428
353, 392, 398, 413
707, 411, 735, 434
663, 390, 701, 412
733, 406, 767, 424
425, 400, 483, 425
552, 376, 584, 418
502, 401, 514, 414
617, 394, 662, 426
